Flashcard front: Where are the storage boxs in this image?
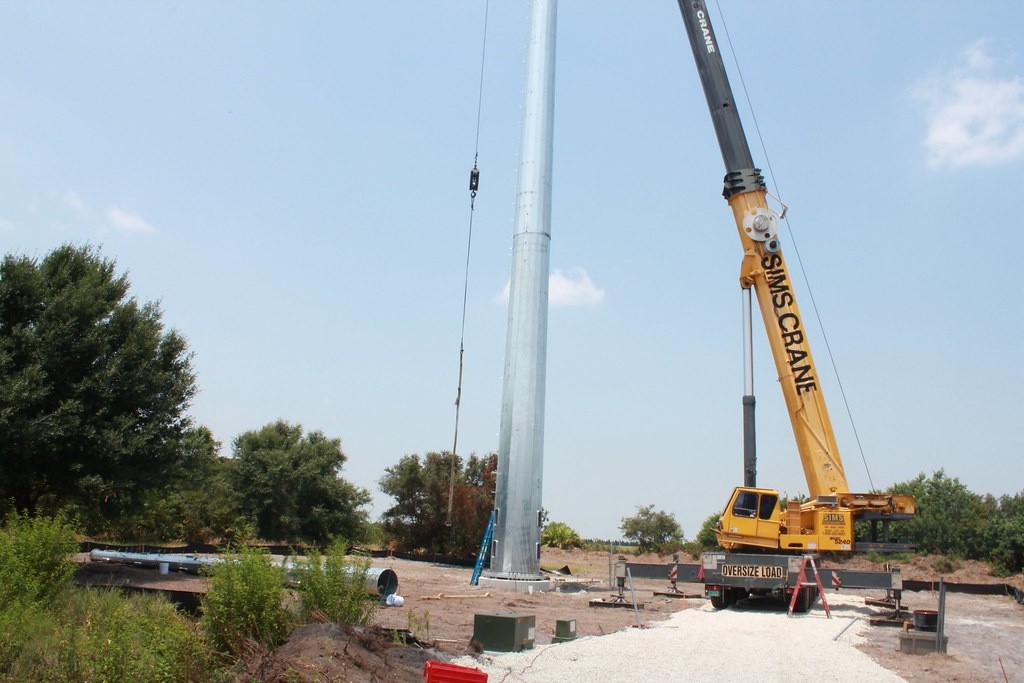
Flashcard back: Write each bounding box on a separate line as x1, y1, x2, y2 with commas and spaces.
424, 661, 488, 683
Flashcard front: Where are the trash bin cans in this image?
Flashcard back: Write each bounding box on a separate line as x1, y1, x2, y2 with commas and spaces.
423, 660, 488, 683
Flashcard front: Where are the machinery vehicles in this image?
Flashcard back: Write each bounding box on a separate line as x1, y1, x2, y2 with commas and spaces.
444, 1, 919, 625
